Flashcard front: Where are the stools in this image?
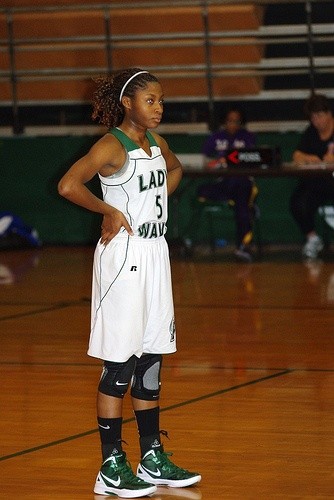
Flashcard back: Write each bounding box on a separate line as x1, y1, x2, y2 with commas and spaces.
189, 196, 261, 262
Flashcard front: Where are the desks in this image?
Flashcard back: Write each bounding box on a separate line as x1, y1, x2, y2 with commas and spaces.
182, 163, 333, 178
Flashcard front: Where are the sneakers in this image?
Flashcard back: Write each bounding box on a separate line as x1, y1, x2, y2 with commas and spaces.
94, 451, 157, 498
137, 445, 201, 488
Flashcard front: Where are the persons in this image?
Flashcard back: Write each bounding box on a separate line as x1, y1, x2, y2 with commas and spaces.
57, 67, 202, 499
165, 109, 259, 264
284, 95, 334, 258
